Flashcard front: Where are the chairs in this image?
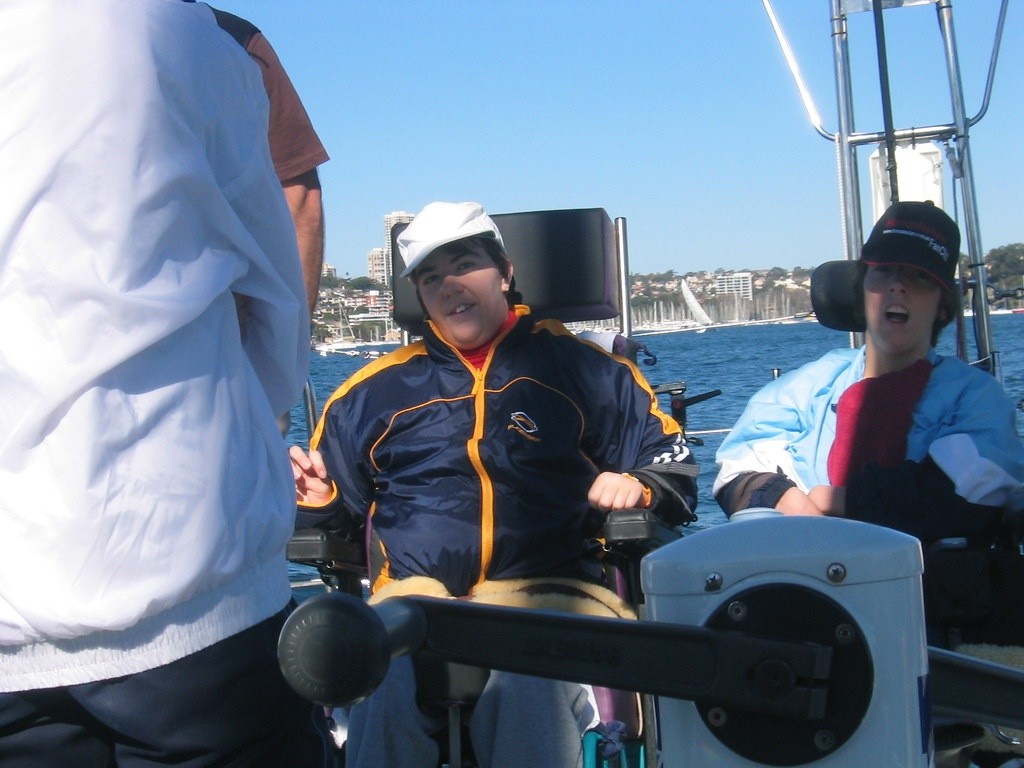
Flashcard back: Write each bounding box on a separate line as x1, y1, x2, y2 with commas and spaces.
812, 261, 1024, 648
284, 206, 691, 768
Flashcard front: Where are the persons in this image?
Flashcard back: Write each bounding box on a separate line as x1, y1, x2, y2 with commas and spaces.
0, 1, 332, 768
708, 200, 1024, 645
283, 201, 701, 768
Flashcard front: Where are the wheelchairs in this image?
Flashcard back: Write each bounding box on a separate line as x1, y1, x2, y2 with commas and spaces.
282, 208, 721, 768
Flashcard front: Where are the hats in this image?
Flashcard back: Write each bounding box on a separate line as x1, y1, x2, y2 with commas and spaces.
396, 202, 504, 278
862, 201, 960, 296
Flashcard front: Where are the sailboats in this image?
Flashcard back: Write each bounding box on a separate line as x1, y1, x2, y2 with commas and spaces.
315, 299, 358, 351
568, 278, 714, 336
